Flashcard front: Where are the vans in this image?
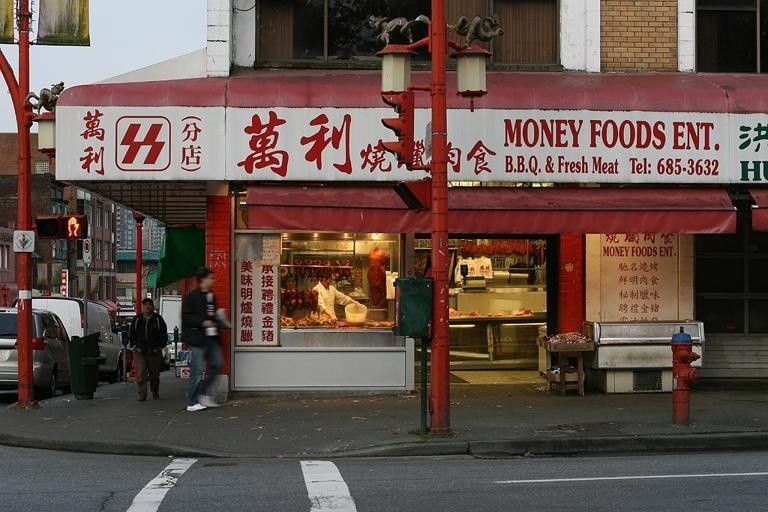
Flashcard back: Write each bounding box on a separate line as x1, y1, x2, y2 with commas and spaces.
14, 297, 124, 384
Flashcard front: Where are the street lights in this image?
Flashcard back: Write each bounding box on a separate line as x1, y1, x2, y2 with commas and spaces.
0, 284, 10, 305
376, 34, 491, 435
132, 211, 147, 315
83, 244, 126, 335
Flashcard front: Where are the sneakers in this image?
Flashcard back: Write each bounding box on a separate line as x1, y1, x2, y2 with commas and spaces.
139, 393, 158, 402
201, 397, 218, 408
187, 403, 207, 412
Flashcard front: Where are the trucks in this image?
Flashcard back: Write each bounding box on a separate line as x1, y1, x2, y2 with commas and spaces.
160, 295, 184, 361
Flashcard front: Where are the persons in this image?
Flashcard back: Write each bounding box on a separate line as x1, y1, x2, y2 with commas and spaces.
128, 298, 169, 402
179, 268, 227, 410
284, 273, 298, 288
311, 276, 362, 324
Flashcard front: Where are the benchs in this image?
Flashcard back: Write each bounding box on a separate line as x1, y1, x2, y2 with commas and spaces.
693, 332, 768, 381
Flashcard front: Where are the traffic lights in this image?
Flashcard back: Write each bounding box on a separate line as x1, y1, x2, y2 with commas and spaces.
381, 91, 414, 165
391, 180, 431, 209
36, 213, 88, 241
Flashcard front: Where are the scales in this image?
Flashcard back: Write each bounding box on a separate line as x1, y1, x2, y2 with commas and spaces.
460, 264, 486, 289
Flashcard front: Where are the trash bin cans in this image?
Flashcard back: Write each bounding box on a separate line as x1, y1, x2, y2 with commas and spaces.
68, 331, 103, 400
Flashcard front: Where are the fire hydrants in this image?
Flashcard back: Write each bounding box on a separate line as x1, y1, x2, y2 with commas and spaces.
669, 326, 701, 427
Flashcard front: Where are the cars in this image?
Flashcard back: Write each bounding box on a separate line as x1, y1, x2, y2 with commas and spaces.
0, 306, 73, 400
97, 299, 171, 371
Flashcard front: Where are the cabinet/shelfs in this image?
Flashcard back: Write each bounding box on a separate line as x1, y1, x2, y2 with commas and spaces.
537, 336, 594, 398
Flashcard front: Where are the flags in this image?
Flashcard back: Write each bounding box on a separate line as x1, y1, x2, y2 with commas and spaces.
36, 0, 90, 47
0, 1, 15, 45
